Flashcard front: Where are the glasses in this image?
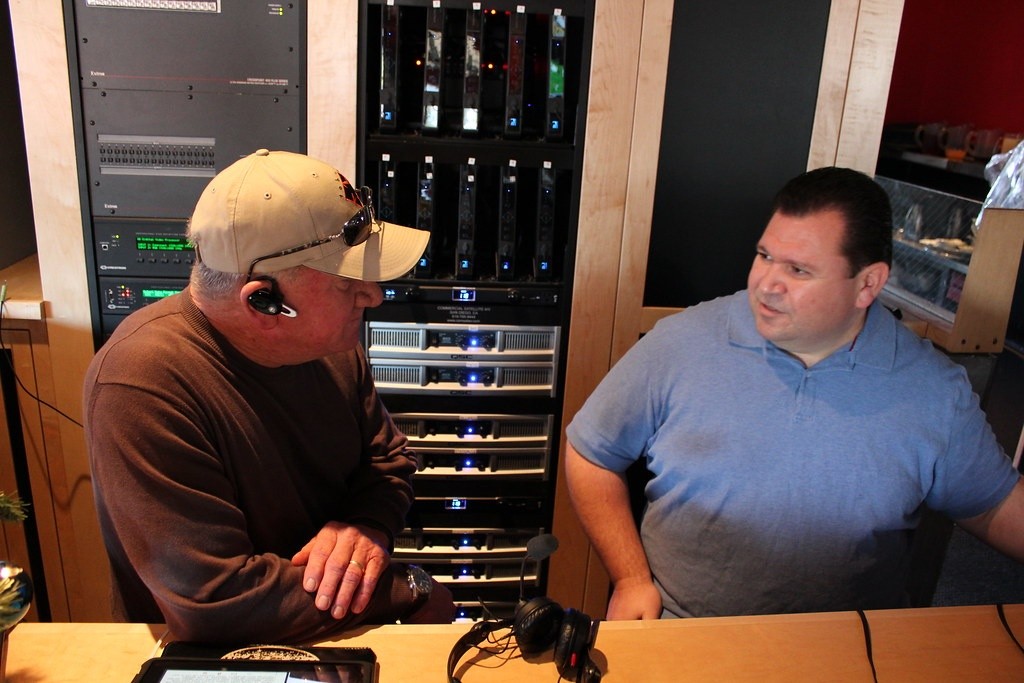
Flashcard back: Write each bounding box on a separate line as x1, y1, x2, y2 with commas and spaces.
245, 185, 373, 286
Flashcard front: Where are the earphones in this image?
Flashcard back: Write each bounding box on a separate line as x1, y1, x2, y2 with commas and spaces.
248, 290, 297, 319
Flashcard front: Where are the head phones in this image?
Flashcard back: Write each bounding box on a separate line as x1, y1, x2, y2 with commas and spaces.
448, 596, 601, 683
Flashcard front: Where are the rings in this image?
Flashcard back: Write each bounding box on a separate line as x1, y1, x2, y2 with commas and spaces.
349, 560, 365, 573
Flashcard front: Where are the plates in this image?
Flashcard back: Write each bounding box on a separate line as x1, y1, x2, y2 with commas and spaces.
919, 241, 972, 263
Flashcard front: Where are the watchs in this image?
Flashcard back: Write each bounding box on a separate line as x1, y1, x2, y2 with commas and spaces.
394, 562, 431, 622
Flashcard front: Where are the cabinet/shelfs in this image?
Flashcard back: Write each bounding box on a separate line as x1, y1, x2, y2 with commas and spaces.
870, 157, 1024, 356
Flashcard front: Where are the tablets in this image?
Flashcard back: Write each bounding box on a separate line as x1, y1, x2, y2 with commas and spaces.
131, 656, 375, 683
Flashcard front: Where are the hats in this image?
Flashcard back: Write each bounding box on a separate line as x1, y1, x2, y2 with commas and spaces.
190, 148, 430, 283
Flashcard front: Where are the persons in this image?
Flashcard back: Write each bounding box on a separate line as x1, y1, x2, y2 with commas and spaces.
564, 167, 1023, 621
82, 149, 458, 641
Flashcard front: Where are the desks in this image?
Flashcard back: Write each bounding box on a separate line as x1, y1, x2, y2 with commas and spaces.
6, 599, 1023, 683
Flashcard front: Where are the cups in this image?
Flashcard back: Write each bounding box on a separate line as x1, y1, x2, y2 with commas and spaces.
938, 125, 967, 152
965, 130, 998, 166
992, 133, 1022, 158
914, 123, 939, 154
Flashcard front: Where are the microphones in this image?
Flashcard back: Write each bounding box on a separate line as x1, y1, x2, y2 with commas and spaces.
519, 532, 559, 602
